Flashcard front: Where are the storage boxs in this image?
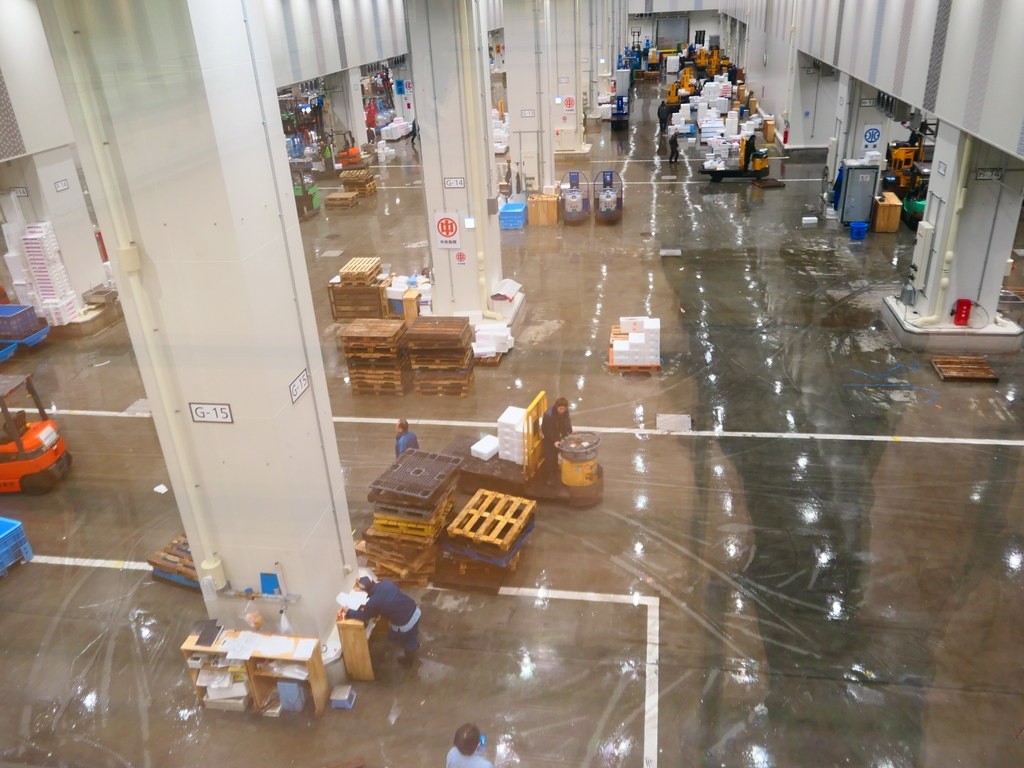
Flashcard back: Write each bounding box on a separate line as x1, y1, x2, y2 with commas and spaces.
276, 677, 308, 713
0, 190, 82, 365
186, 652, 208, 668
491, 109, 509, 154
206, 680, 249, 699
850, 221, 868, 240
802, 217, 818, 224
87, 289, 118, 307
648, 43, 775, 169
262, 687, 282, 717
203, 692, 250, 711
500, 203, 527, 230
295, 183, 321, 216
0, 516, 33, 578
376, 116, 412, 161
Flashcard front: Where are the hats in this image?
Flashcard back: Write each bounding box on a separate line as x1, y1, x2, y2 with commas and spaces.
359, 577, 371, 591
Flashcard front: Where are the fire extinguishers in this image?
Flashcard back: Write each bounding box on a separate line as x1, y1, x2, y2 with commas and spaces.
955, 298, 989, 330
783, 130, 788, 144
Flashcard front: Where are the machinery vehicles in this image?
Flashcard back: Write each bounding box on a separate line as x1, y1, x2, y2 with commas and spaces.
325, 129, 369, 178
664, 46, 730, 115
882, 115, 933, 232
0, 373, 72, 498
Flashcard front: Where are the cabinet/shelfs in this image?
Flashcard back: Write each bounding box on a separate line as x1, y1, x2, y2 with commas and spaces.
338, 612, 389, 682
872, 192, 904, 233
527, 194, 559, 225
749, 97, 756, 116
180, 629, 329, 717
762, 120, 774, 142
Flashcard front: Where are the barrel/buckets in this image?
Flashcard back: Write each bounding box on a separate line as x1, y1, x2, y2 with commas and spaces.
850, 222, 867, 239
557, 431, 601, 488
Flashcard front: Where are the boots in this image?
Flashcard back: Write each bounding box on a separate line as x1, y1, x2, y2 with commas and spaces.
397, 650, 417, 666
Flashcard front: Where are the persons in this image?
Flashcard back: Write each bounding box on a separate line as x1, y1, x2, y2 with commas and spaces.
657, 101, 669, 132
659, 52, 664, 69
689, 44, 695, 51
446, 723, 495, 768
728, 65, 737, 82
542, 397, 572, 487
342, 576, 422, 668
745, 135, 757, 169
367, 127, 375, 142
411, 119, 417, 143
396, 418, 419, 459
669, 130, 680, 164
